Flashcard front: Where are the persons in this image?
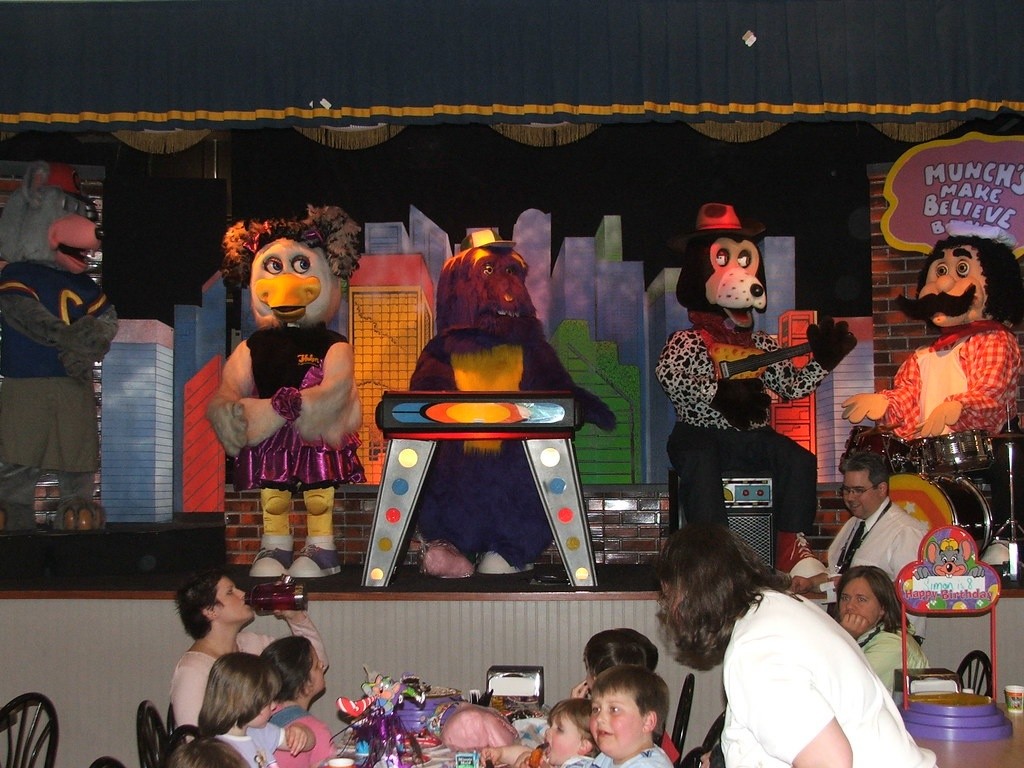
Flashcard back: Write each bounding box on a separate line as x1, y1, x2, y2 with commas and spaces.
169, 569, 328, 730
198, 652, 316, 768
657, 526, 938, 768
836, 565, 929, 699
260, 636, 336, 768
167, 735, 252, 768
790, 453, 926, 644
589, 666, 673, 768
481, 699, 597, 768
570, 628, 658, 699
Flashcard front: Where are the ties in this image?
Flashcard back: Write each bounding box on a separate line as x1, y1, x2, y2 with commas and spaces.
827, 522, 865, 616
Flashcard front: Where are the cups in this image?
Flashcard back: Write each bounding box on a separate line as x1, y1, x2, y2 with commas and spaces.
1004, 685, 1024, 713
329, 758, 354, 768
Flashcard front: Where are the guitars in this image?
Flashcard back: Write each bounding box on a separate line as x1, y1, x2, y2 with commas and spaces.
707, 332, 855, 379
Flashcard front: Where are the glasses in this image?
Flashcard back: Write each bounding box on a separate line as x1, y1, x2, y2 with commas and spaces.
838, 480, 878, 497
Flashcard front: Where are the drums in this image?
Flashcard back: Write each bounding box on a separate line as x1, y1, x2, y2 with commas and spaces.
837, 425, 911, 481
911, 429, 995, 473
887, 473, 993, 563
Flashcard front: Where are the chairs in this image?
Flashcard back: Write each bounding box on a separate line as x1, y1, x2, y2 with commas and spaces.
0, 693, 200, 768
671, 674, 726, 768
956, 648, 993, 699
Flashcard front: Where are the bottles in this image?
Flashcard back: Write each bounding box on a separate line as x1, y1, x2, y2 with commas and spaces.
244, 584, 305, 611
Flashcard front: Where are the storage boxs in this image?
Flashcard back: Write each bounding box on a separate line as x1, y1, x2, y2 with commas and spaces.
485, 665, 544, 709
894, 668, 961, 695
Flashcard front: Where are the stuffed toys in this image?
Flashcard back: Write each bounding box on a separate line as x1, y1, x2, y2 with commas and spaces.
407, 229, 616, 577
654, 203, 857, 577
0, 161, 117, 534
842, 235, 1023, 567
207, 207, 363, 576
